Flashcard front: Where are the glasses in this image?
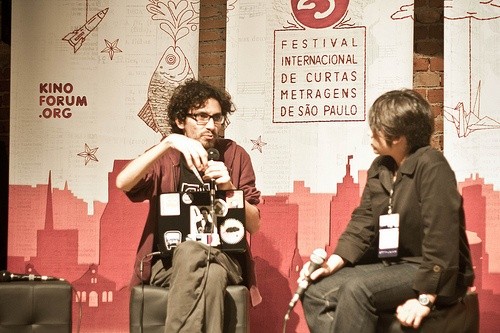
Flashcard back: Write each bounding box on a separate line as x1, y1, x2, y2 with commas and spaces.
184, 111, 225, 126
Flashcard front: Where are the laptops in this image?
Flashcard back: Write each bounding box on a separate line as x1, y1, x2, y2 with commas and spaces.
157, 189, 249, 288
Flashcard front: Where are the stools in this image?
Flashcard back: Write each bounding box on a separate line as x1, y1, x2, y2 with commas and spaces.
0, 281, 479, 333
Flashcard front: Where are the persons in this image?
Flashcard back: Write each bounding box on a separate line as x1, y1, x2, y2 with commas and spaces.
195, 207, 214, 233
114, 80, 262, 333
295, 88, 474, 333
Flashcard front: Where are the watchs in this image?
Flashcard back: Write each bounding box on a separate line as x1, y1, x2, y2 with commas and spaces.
417, 294, 436, 311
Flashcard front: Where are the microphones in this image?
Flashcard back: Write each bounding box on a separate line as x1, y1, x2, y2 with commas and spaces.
0, 271, 60, 283
287, 247, 327, 311
206, 148, 220, 202
